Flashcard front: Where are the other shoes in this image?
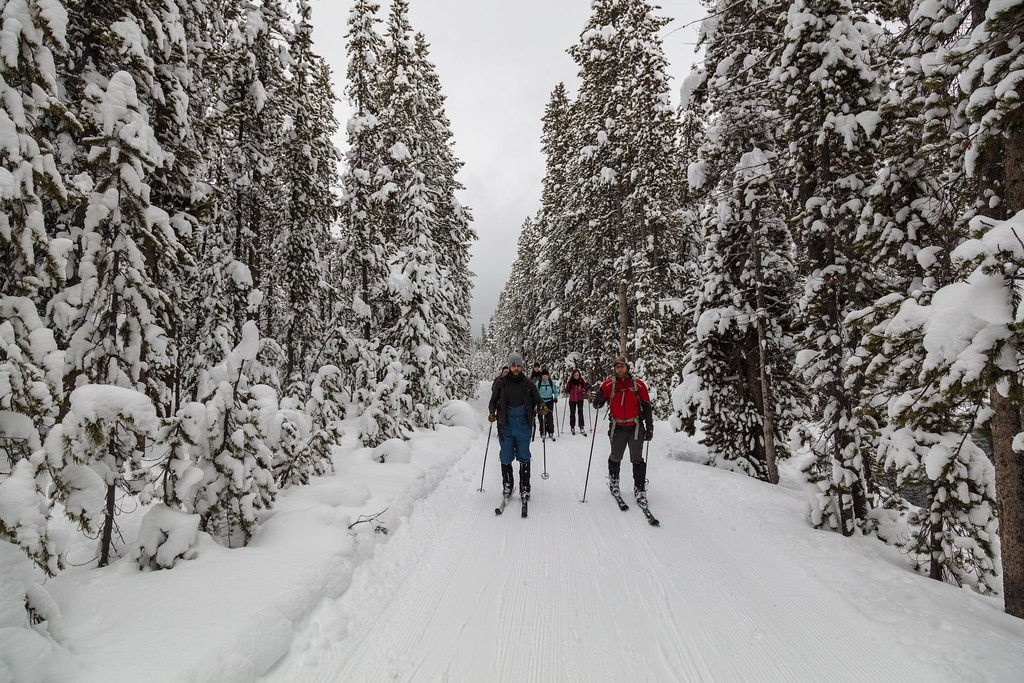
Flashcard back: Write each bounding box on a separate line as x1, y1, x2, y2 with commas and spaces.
571, 426, 575, 432
549, 433, 553, 437
541, 434, 546, 437
580, 427, 585, 433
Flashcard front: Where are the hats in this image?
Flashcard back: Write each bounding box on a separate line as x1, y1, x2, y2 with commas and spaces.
613, 356, 629, 367
508, 352, 522, 368
541, 369, 550, 376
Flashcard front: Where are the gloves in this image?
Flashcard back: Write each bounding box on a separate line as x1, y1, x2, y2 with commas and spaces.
594, 390, 604, 403
643, 425, 653, 441
488, 413, 497, 422
538, 405, 548, 415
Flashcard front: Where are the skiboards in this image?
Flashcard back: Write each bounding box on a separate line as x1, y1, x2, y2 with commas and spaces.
607, 480, 659, 526
572, 431, 587, 437
542, 435, 556, 443
495, 494, 528, 518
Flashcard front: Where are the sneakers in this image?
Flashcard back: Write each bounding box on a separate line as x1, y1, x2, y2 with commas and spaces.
634, 486, 648, 503
609, 477, 620, 492
503, 482, 514, 498
520, 484, 531, 499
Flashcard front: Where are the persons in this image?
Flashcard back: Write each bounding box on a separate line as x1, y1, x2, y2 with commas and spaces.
492, 366, 510, 437
592, 356, 654, 509
529, 362, 558, 438
564, 369, 588, 434
488, 353, 549, 501
